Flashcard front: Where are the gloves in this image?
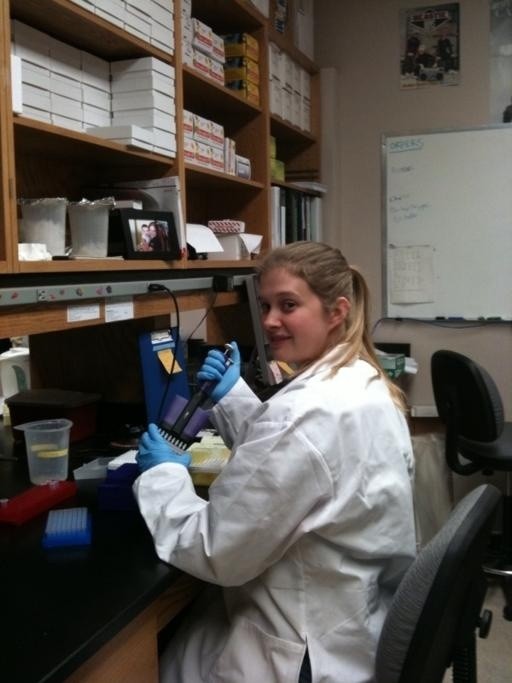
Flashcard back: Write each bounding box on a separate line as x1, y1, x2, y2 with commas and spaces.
197, 341, 241, 401
135, 423, 191, 474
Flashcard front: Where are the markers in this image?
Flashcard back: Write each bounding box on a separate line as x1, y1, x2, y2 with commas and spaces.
435, 316, 502, 321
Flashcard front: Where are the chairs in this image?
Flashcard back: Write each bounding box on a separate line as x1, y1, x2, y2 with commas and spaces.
432, 350, 512, 623
374, 481, 501, 683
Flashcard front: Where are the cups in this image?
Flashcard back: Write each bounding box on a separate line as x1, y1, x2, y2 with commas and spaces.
22, 194, 111, 258
15, 418, 74, 486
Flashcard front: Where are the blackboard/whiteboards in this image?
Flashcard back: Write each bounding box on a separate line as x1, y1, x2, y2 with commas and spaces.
380, 122, 512, 323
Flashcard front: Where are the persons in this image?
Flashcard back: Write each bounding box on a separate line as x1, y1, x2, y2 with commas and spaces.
132, 242, 417, 683
139, 224, 150, 251
148, 222, 168, 251
